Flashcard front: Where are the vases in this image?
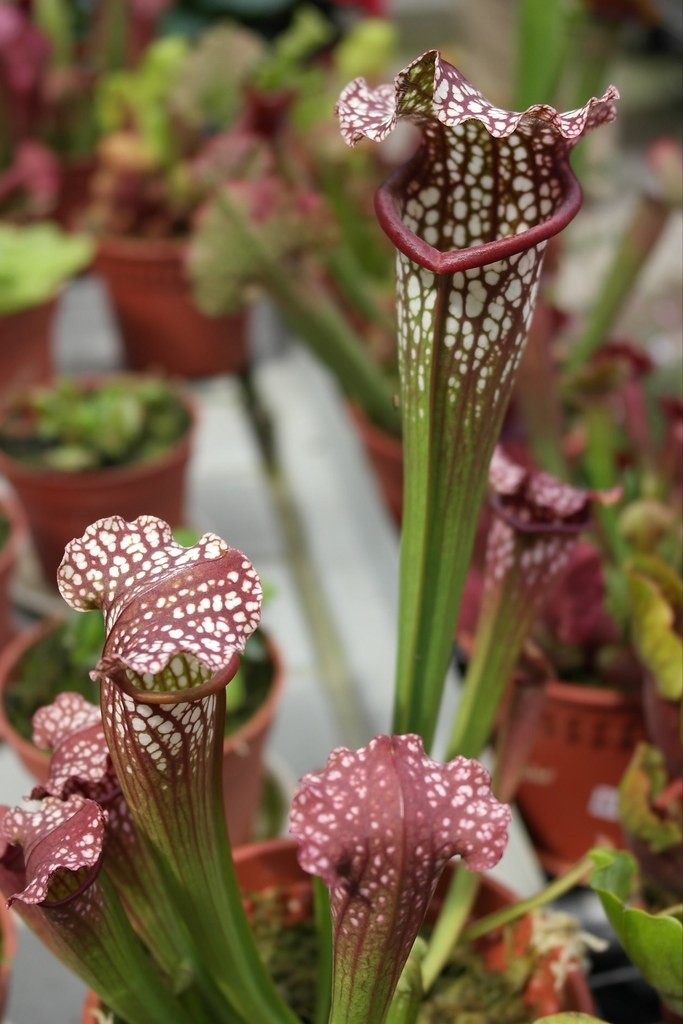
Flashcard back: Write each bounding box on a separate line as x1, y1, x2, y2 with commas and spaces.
81, 837, 597, 1024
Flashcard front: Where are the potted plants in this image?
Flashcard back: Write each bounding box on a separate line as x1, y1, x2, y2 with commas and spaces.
0, 1, 683, 895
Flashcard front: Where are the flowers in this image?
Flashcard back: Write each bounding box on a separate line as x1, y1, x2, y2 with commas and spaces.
1, 48, 683, 1024
